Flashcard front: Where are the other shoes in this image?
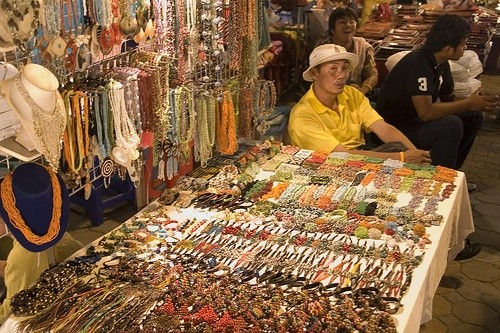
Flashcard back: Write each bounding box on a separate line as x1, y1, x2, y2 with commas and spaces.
452, 237, 482, 260
467, 182, 477, 192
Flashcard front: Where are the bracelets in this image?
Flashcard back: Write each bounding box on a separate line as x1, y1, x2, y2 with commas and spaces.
363, 84, 373, 92
91, 206, 208, 267
325, 209, 380, 225
400, 152, 404, 162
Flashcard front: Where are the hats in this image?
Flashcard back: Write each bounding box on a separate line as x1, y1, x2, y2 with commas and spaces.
303, 43, 359, 81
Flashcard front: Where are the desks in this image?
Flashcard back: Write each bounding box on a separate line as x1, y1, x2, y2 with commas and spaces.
0, 139, 476, 333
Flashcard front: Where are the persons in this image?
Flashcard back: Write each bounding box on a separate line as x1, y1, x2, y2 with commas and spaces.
366, 15, 500, 192
0, 231, 85, 324
288, 44, 482, 260
313, 7, 381, 109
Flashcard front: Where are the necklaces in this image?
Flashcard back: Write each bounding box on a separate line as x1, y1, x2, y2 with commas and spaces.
0, 0, 278, 252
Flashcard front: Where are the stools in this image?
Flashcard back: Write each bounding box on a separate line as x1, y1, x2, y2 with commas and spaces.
63, 156, 134, 225
144, 140, 194, 199
